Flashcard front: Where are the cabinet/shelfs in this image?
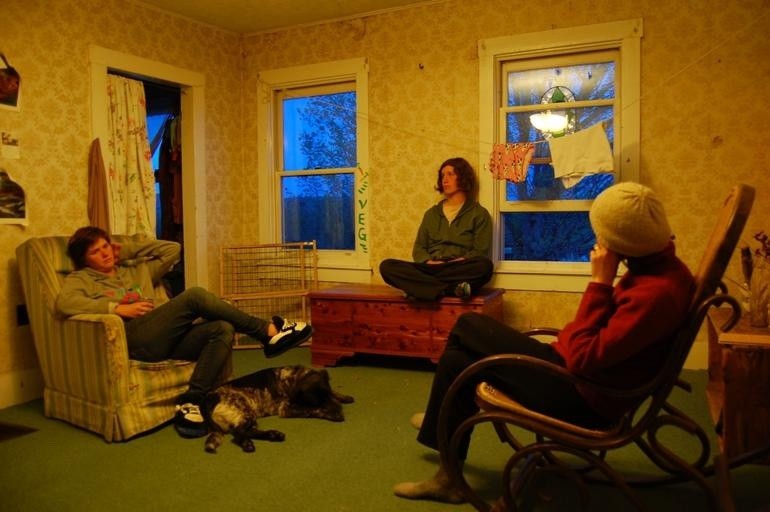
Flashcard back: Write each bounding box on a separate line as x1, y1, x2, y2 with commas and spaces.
307, 281, 505, 371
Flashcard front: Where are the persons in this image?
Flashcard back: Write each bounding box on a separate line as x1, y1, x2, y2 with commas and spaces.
376, 155, 496, 308
53, 222, 317, 439
390, 179, 698, 502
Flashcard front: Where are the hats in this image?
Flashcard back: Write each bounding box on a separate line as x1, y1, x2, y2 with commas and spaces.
590, 181, 672, 255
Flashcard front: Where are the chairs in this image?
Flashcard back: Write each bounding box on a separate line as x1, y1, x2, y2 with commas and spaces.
15, 231, 236, 446
436, 184, 764, 512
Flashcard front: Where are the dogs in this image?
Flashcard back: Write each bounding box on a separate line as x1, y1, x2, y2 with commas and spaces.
198, 364, 354, 454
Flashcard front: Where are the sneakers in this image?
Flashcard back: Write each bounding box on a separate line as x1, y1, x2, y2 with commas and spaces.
174, 393, 210, 437
454, 281, 471, 298
264, 315, 313, 359
